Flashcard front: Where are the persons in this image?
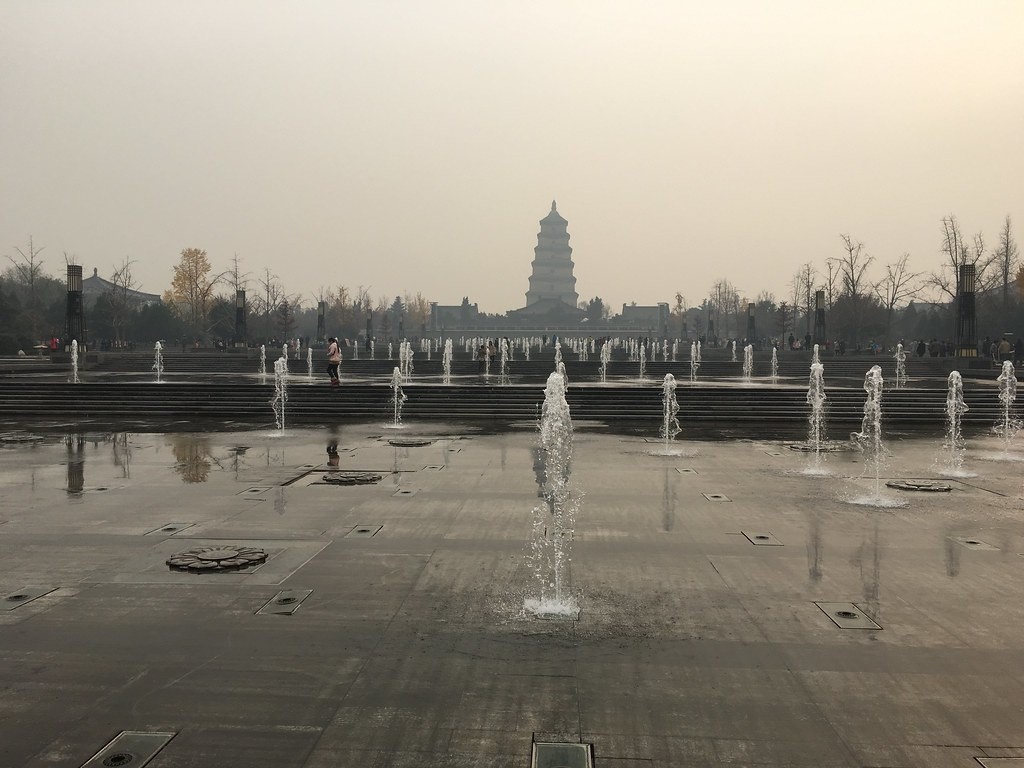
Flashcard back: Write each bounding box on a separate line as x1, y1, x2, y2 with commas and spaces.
175, 338, 180, 347
325, 438, 341, 476
503, 337, 511, 357
50, 336, 57, 352
982, 335, 1011, 363
644, 337, 648, 349
588, 335, 593, 350
213, 339, 226, 353
869, 338, 878, 356
282, 336, 303, 348
542, 334, 549, 348
1013, 338, 1024, 367
194, 338, 201, 349
486, 340, 497, 365
92, 334, 97, 350
476, 344, 487, 374
366, 337, 373, 352
595, 336, 612, 352
410, 335, 421, 353
389, 337, 400, 358
700, 332, 861, 357
326, 338, 341, 384
638, 335, 643, 351
494, 338, 499, 353
915, 337, 947, 357
58, 337, 63, 349
306, 335, 310, 349
552, 333, 558, 347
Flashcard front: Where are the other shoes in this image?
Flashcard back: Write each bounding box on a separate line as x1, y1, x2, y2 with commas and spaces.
332, 380, 338, 385
